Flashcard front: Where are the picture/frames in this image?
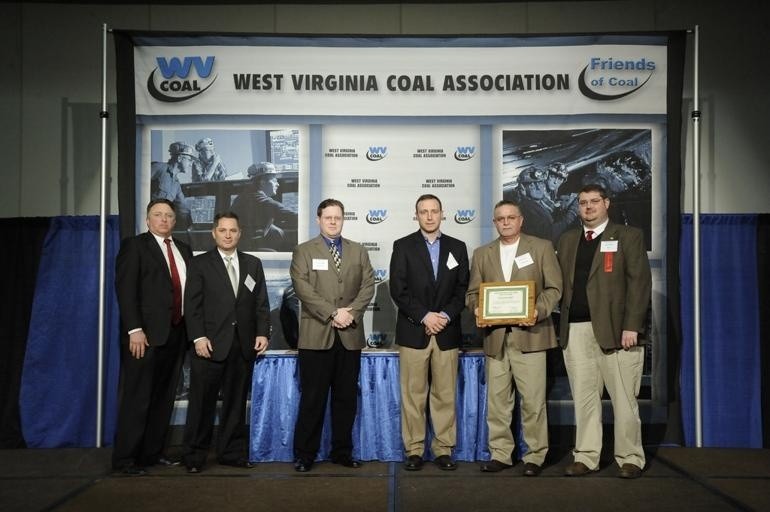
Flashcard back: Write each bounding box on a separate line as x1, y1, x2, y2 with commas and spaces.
475, 280, 538, 327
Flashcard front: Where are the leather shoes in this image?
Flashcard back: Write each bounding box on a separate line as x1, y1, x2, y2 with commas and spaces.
406, 454, 424, 470
294, 459, 313, 472
480, 460, 645, 479
219, 459, 253, 467
114, 457, 200, 476
434, 456, 456, 470
332, 455, 359, 468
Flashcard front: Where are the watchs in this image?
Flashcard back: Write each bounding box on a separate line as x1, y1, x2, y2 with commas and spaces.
332, 309, 339, 318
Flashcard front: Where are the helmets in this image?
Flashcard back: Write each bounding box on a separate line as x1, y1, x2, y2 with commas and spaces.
169, 139, 213, 161
248, 161, 282, 176
516, 162, 569, 183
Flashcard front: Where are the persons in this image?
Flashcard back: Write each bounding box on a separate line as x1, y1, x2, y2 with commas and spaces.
547, 159, 580, 210
193, 137, 228, 181
288, 198, 374, 472
268, 286, 288, 350
555, 183, 652, 479
465, 201, 564, 477
280, 282, 299, 350
151, 142, 198, 228
514, 168, 580, 239
109, 198, 193, 476
390, 194, 471, 472
184, 212, 271, 473
226, 162, 297, 252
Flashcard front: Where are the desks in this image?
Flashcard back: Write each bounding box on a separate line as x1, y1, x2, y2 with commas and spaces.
248, 348, 525, 467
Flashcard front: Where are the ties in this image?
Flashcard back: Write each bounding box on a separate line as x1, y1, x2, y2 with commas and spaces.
164, 240, 182, 324
586, 231, 593, 241
224, 256, 238, 299
329, 241, 342, 274
